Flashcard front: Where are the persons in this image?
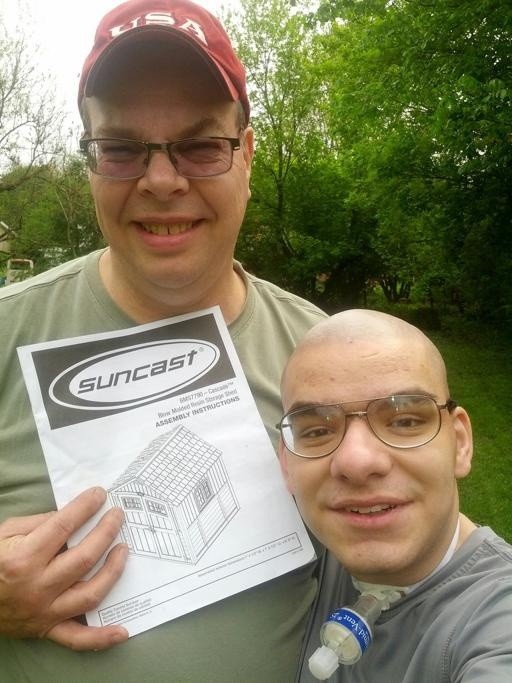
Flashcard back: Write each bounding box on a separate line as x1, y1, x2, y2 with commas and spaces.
276, 306, 511, 683
1, 0, 335, 683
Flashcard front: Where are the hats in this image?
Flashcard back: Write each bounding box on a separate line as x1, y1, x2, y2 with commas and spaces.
76, 0, 251, 104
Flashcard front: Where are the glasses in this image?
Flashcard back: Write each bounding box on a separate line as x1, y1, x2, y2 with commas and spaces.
274, 389, 456, 459
77, 119, 243, 180
